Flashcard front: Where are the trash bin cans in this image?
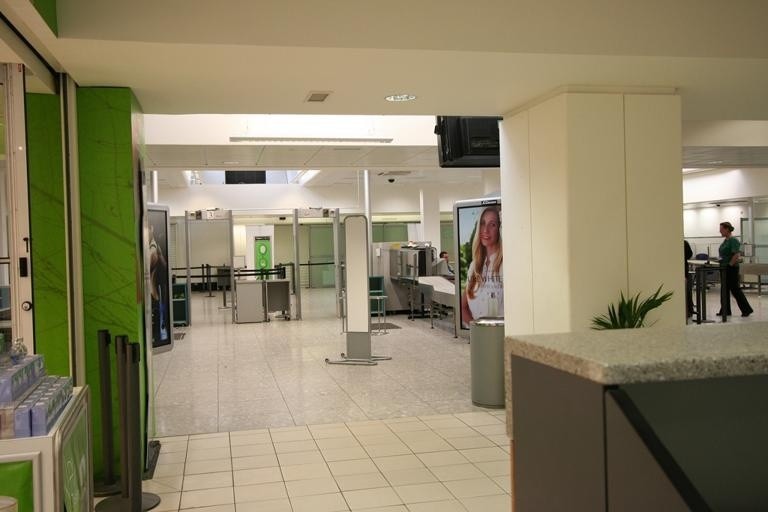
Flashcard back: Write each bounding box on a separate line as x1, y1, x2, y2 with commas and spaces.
172, 282, 188, 327
276, 263, 294, 296
217, 269, 230, 291
469, 316, 504, 408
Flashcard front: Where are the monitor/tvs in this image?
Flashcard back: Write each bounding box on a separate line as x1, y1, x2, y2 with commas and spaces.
434, 115, 501, 169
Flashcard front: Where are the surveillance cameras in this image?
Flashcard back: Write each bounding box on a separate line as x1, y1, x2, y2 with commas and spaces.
388, 179, 394, 183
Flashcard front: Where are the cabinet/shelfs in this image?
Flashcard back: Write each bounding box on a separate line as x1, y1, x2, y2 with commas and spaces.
0, 384, 95, 512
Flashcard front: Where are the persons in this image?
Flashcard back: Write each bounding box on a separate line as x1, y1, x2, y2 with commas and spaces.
146, 213, 168, 346
715, 221, 754, 319
460, 206, 505, 328
683, 239, 694, 318
439, 251, 455, 276
